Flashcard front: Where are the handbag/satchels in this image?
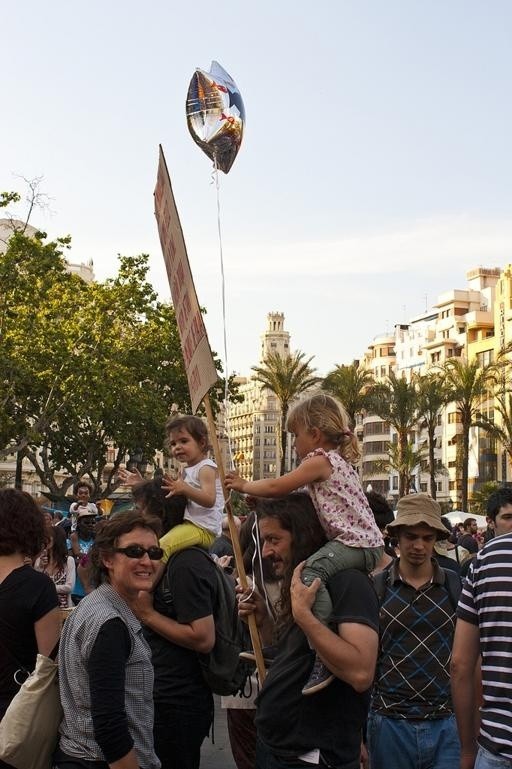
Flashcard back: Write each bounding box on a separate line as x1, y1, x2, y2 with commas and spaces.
0, 634, 69, 769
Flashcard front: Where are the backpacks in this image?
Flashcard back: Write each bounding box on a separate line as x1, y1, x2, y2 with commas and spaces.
168, 546, 246, 695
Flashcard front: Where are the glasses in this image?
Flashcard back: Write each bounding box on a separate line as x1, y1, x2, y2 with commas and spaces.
113, 543, 164, 561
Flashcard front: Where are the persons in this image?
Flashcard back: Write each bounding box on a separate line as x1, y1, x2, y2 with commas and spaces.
461, 484, 511, 586
451, 533, 512, 769
366, 496, 462, 769
130, 478, 216, 769
225, 395, 383, 693
58, 513, 164, 769
210, 515, 241, 565
435, 516, 468, 560
237, 490, 366, 769
1, 489, 60, 769
67, 512, 97, 604
34, 526, 76, 611
53, 510, 66, 528
71, 482, 103, 555
367, 490, 395, 597
219, 497, 285, 769
481, 521, 495, 541
119, 417, 225, 564
462, 517, 479, 554
43, 513, 52, 526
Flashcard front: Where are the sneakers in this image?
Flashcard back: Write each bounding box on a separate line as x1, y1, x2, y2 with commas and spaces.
238, 644, 276, 664
302, 651, 335, 694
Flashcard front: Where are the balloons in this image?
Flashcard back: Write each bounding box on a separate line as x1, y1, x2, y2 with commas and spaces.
187, 62, 244, 173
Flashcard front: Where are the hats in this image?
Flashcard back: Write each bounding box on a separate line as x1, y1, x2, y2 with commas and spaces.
77, 507, 97, 518
385, 493, 449, 542
